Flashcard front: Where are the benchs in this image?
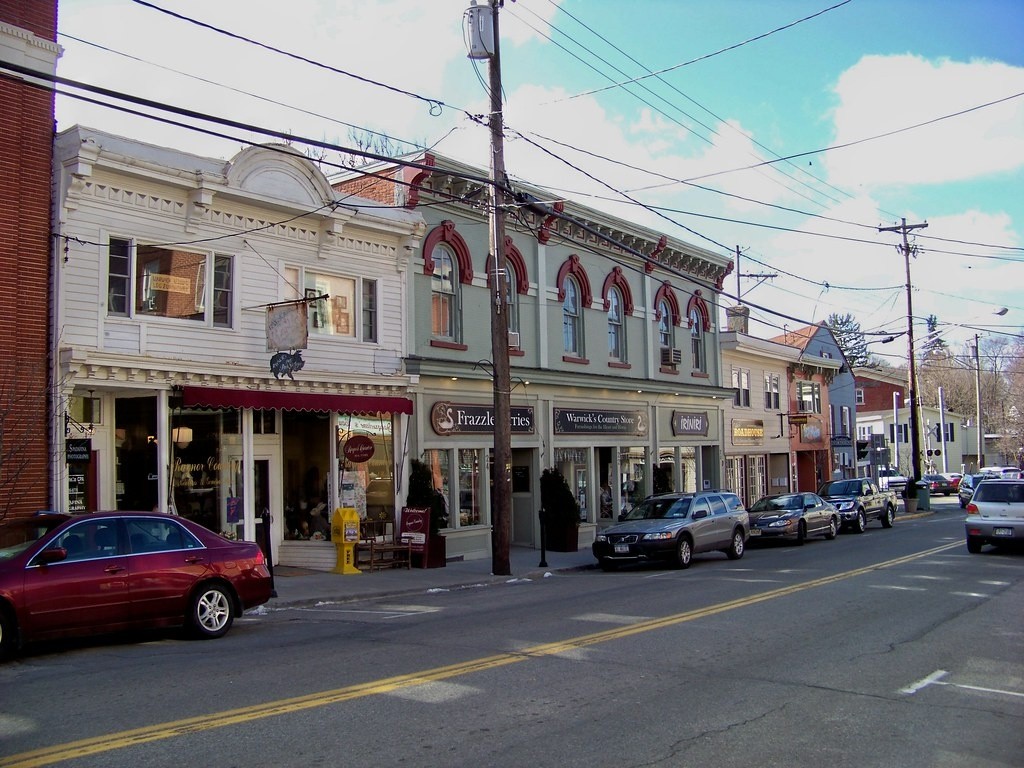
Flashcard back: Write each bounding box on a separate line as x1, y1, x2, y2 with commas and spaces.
355, 518, 415, 573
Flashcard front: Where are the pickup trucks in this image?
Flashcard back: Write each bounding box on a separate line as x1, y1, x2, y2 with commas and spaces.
817, 478, 899, 532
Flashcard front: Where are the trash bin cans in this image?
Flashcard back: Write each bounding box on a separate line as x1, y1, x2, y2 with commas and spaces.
915, 480, 931, 511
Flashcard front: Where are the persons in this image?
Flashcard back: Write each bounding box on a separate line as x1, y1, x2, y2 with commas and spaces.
600, 484, 613, 519
62, 535, 95, 561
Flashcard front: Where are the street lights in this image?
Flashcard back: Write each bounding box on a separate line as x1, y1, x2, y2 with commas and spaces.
880, 306, 1008, 479
893, 392, 901, 470
939, 404, 955, 472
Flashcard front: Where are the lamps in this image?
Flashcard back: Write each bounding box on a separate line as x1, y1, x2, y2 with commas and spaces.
65, 411, 97, 439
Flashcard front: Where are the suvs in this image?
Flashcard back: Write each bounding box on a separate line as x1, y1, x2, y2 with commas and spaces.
958, 474, 1012, 508
591, 490, 751, 569
878, 467, 908, 497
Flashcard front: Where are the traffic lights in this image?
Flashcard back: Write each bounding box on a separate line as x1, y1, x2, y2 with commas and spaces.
856, 441, 869, 459
935, 449, 942, 456
927, 449, 932, 456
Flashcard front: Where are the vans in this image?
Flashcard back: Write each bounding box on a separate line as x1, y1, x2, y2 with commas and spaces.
979, 467, 1024, 479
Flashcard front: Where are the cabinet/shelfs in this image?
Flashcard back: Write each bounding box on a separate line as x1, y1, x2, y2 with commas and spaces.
458, 470, 479, 493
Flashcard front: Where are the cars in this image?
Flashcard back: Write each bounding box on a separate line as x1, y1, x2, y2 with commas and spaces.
964, 479, 1024, 553
939, 473, 963, 491
920, 474, 951, 496
0, 510, 277, 652
746, 493, 841, 541
